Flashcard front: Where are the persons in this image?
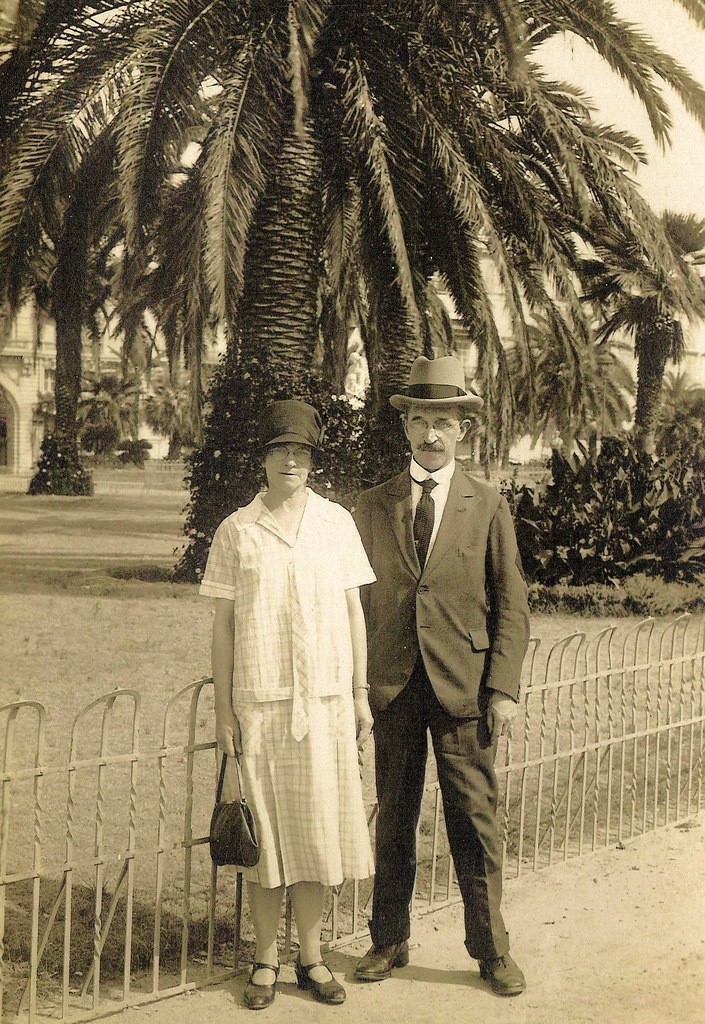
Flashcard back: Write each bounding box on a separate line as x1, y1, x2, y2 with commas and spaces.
197, 399, 378, 1009
351, 355, 531, 994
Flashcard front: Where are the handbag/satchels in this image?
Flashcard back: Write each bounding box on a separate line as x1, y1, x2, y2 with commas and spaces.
211, 748, 262, 869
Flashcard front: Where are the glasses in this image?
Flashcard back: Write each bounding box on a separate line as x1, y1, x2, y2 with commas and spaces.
406, 416, 460, 435
263, 447, 312, 463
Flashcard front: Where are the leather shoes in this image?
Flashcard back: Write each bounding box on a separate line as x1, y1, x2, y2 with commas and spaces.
295, 953, 347, 1004
244, 956, 280, 1009
354, 939, 410, 980
478, 953, 527, 994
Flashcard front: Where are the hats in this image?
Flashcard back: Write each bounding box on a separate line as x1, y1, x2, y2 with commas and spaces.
256, 400, 324, 453
389, 356, 485, 413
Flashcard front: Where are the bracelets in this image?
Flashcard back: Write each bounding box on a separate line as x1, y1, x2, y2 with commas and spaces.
353, 684, 371, 692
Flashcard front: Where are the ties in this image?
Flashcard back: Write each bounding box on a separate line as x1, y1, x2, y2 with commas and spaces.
409, 473, 439, 572
